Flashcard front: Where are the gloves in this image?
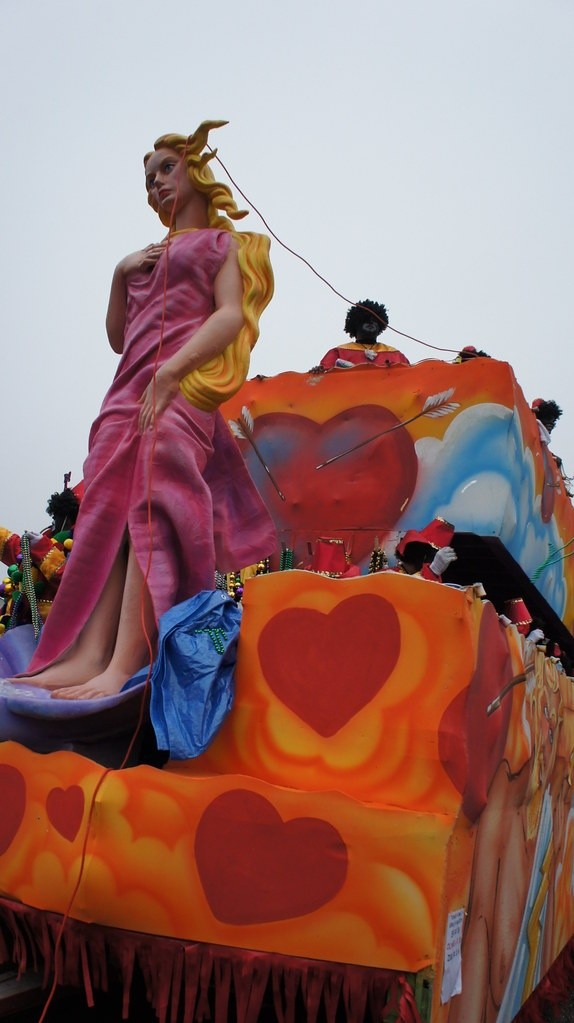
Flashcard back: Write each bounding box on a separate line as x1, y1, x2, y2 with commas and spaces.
429, 546, 457, 576
527, 629, 545, 643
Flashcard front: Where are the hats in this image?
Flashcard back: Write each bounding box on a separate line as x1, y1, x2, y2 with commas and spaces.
504, 598, 532, 625
396, 516, 456, 557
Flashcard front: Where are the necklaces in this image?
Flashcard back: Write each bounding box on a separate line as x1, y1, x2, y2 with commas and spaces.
4, 534, 44, 642
212, 557, 270, 607
302, 536, 360, 578
279, 549, 294, 571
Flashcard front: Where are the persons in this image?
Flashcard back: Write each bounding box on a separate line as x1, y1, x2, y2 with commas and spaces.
532, 398, 563, 470
508, 597, 545, 644
375, 515, 458, 583
308, 299, 410, 375
9, 118, 277, 702
0, 471, 87, 602
